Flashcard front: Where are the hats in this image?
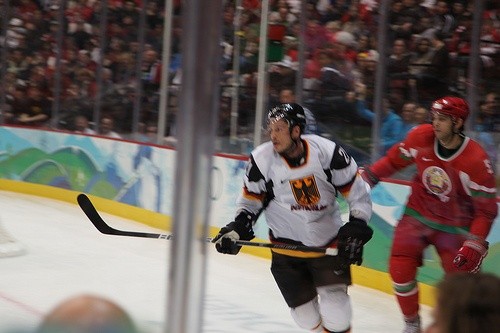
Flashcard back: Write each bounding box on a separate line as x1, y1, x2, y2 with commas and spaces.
8, 17, 24, 27
480, 34, 495, 43
331, 30, 358, 48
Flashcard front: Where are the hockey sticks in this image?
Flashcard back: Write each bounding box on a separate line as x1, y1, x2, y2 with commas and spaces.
76, 193, 339, 257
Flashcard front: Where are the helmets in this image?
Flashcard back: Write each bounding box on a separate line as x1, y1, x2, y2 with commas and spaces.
431, 96, 470, 121
267, 102, 306, 131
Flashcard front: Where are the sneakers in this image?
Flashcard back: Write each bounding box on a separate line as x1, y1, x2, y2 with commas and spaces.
402, 316, 420, 333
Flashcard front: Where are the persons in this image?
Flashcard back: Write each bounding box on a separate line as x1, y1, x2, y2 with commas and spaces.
0, 0, 500, 179
358, 97, 498, 333
36, 296, 136, 333
211, 103, 373, 333
423, 272, 500, 333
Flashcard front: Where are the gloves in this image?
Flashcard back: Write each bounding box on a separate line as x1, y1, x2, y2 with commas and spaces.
358, 166, 378, 189
336, 219, 374, 266
211, 212, 255, 255
452, 240, 489, 276
